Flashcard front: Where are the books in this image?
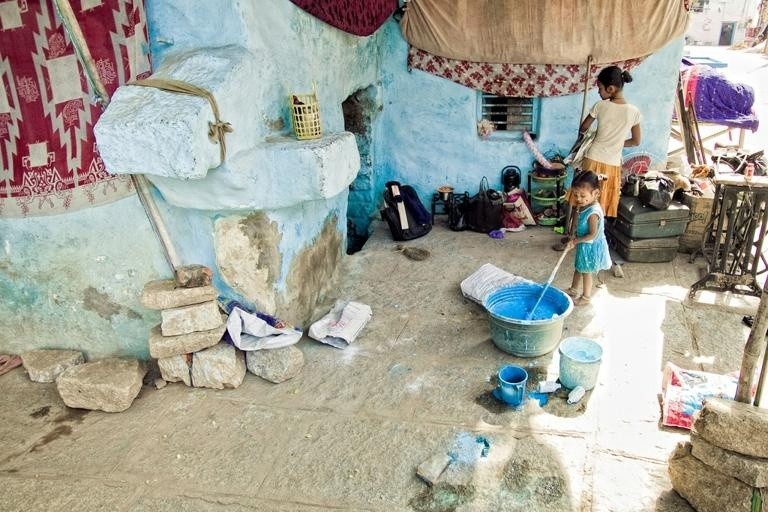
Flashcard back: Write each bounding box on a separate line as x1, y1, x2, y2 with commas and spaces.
677, 190, 729, 255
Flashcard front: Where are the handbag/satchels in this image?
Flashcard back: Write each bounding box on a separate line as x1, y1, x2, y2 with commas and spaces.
574, 238, 579, 245
583, 235, 588, 241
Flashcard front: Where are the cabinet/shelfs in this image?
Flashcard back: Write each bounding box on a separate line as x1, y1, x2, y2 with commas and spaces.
566, 387, 585, 404
744, 162, 755, 182
478, 175, 489, 198
538, 380, 563, 393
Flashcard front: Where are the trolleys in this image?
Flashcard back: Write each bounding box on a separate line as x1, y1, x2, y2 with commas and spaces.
621, 149, 663, 192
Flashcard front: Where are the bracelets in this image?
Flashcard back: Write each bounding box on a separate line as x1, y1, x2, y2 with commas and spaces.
481, 283, 574, 356
537, 160, 567, 178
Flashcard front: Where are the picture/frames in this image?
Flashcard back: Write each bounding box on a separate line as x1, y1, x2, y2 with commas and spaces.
431, 192, 469, 226
685, 163, 767, 305
661, 108, 760, 171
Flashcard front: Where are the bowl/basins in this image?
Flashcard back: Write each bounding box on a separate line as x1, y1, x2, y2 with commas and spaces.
611, 96, 625, 99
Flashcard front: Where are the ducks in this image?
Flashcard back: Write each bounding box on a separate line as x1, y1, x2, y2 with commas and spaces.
604, 223, 679, 262
607, 197, 691, 239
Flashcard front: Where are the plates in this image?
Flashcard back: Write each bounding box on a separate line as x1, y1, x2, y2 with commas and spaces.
525, 170, 570, 228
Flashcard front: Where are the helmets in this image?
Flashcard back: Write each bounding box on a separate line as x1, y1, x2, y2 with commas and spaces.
435, 188, 454, 193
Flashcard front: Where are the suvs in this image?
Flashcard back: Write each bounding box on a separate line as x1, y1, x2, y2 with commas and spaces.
498, 365, 530, 408
557, 335, 604, 392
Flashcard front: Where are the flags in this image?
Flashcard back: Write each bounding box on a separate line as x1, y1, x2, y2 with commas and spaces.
436, 186, 455, 201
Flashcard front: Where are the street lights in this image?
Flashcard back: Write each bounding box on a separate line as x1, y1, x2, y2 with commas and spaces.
380, 181, 432, 241
447, 187, 537, 234
712, 150, 768, 176
620, 170, 675, 210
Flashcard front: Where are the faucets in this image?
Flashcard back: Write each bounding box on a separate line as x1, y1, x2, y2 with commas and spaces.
563, 288, 579, 297
573, 295, 591, 306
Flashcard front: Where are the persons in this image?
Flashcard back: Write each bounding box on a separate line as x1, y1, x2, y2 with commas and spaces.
563, 168, 613, 306
552, 65, 641, 251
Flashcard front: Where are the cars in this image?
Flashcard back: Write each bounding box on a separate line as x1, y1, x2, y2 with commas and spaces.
497, 365, 529, 405
